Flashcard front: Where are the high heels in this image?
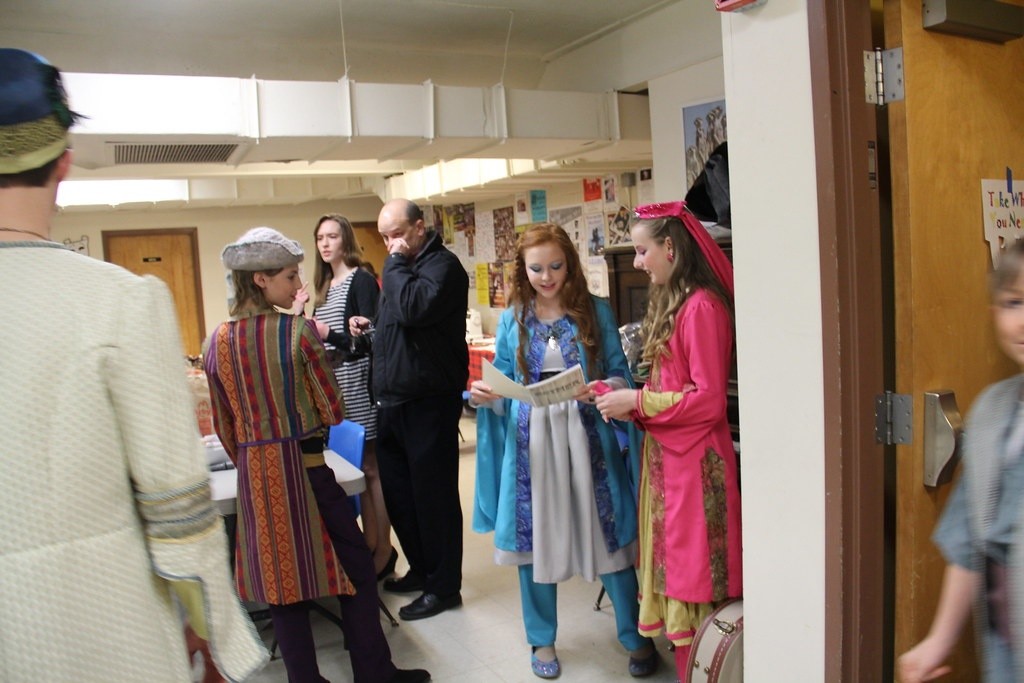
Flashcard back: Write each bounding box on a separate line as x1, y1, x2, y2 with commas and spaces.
375, 545, 398, 581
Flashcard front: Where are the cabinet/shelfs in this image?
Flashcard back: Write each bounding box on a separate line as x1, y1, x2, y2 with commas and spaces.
601, 237, 738, 390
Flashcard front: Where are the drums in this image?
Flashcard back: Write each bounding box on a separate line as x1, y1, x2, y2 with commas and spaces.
682, 598, 743, 683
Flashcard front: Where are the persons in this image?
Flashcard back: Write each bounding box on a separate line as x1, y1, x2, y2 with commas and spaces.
205, 198, 470, 683
897, 237, 1024, 683
0, 47, 266, 683
595, 200, 743, 683
470, 224, 657, 678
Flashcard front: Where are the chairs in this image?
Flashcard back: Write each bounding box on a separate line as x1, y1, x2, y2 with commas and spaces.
328, 419, 399, 628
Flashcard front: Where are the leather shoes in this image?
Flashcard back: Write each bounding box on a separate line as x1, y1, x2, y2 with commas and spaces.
382, 569, 426, 593
398, 588, 462, 620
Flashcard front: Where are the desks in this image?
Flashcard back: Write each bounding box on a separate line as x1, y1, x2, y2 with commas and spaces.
467, 332, 496, 391
210, 449, 366, 663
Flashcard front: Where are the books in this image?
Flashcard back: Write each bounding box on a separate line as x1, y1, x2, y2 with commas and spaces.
481, 356, 586, 408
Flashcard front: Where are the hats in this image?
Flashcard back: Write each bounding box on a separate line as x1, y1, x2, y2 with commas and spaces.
0, 46, 74, 173
221, 227, 305, 270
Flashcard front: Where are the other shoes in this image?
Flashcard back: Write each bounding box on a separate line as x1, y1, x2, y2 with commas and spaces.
530, 644, 560, 678
629, 636, 658, 677
389, 668, 431, 683
